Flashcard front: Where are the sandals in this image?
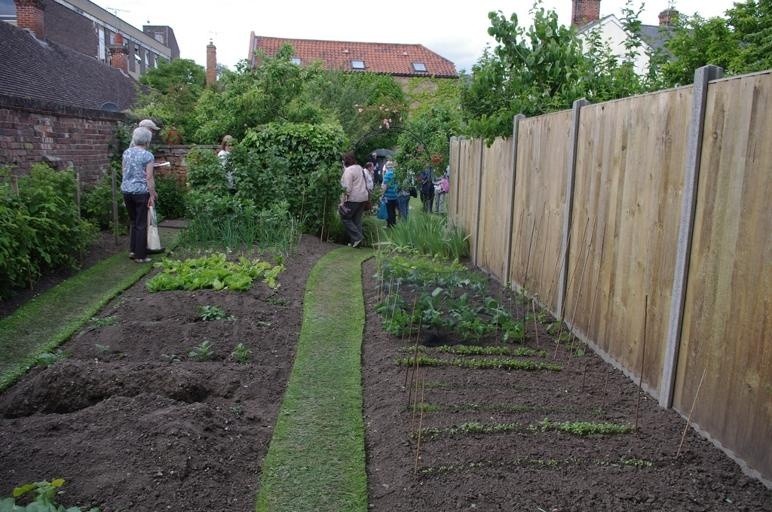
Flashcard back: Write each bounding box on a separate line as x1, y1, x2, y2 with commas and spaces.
128, 252, 152, 263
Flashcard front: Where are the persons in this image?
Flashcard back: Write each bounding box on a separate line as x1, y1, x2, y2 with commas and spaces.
218, 135, 239, 195
338, 152, 450, 248
120, 119, 166, 263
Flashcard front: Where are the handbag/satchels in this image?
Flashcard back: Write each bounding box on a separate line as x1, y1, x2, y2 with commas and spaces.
377, 202, 388, 219
409, 187, 417, 198
440, 175, 449, 193
364, 196, 372, 209
146, 206, 161, 250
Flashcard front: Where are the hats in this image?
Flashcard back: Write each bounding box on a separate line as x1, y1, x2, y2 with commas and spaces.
139, 119, 161, 130
